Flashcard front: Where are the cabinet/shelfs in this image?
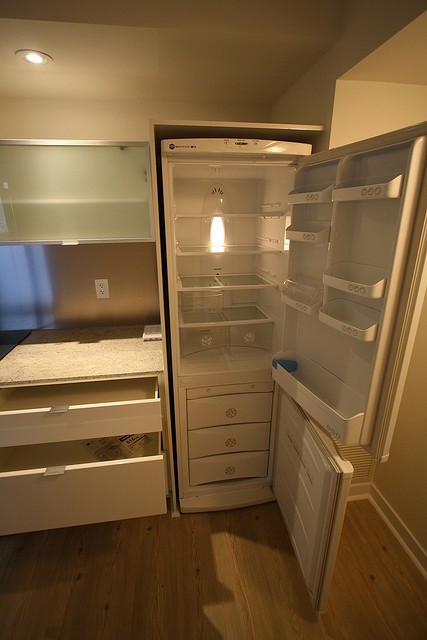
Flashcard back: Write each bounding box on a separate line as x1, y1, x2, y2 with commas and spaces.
1, 141, 156, 245
1, 325, 167, 538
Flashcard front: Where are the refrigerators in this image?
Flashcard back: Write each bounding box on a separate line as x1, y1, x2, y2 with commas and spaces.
152, 120, 427, 617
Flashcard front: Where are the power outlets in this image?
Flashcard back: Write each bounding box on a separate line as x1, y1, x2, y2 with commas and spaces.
93, 278, 112, 301
